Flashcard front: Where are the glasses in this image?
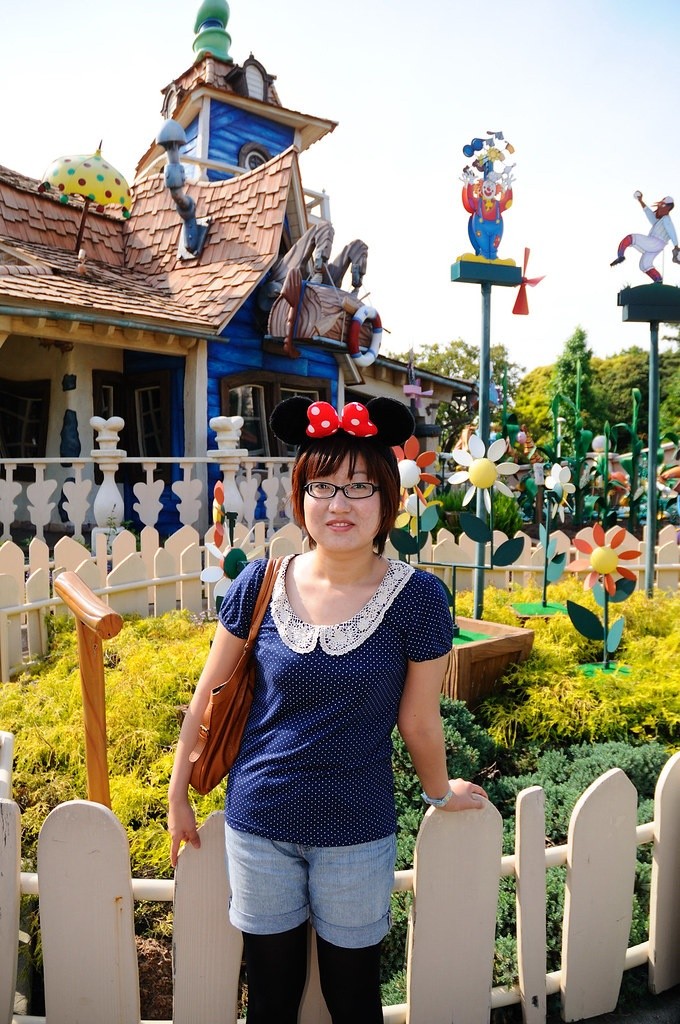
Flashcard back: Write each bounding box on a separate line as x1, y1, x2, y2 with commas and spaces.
304, 479, 379, 500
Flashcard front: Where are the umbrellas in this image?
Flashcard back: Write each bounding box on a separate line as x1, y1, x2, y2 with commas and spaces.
40, 138, 133, 253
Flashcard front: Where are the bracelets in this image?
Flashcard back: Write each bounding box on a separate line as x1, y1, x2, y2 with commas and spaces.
421, 788, 453, 808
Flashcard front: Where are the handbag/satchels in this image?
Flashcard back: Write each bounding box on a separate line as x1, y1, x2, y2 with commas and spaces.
188, 553, 284, 796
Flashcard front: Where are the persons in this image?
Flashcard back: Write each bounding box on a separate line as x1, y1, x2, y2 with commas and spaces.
165, 397, 488, 1024
610, 191, 680, 285
